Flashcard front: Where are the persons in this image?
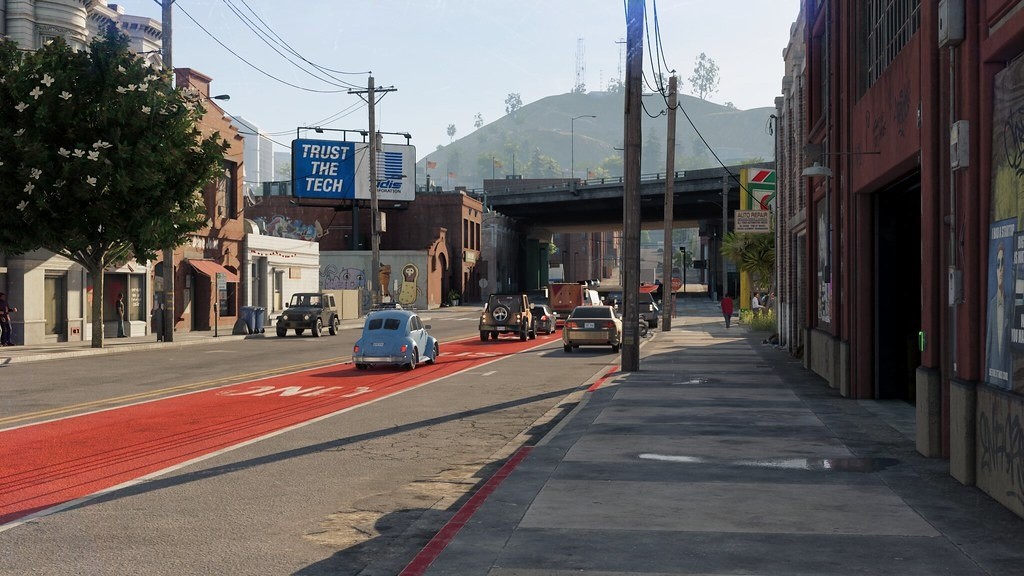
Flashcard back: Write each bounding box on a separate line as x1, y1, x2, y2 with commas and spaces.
654, 279, 663, 303
115, 291, 127, 338
751, 292, 765, 324
0, 293, 18, 346
721, 292, 733, 329
761, 292, 777, 316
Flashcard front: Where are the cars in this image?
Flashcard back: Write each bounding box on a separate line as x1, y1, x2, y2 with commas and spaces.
351, 309, 439, 371
562, 306, 622, 353
529, 304, 556, 334
637, 292, 659, 327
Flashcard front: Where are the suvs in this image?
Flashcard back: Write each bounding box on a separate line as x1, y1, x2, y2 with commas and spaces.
276, 292, 340, 337
479, 294, 536, 342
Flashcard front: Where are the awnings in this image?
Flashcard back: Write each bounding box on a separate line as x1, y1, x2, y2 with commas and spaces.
188, 257, 241, 283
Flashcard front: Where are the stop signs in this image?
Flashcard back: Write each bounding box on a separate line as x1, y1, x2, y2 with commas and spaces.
671, 278, 682, 291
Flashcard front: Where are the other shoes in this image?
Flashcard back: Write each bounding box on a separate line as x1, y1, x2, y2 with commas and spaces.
7, 342, 16, 346
1, 342, 8, 346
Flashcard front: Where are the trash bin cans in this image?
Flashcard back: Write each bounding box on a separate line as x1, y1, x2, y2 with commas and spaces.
255, 307, 266, 334
240, 306, 257, 334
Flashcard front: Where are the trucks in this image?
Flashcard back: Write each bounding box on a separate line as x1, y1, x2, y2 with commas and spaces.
550, 282, 583, 327
